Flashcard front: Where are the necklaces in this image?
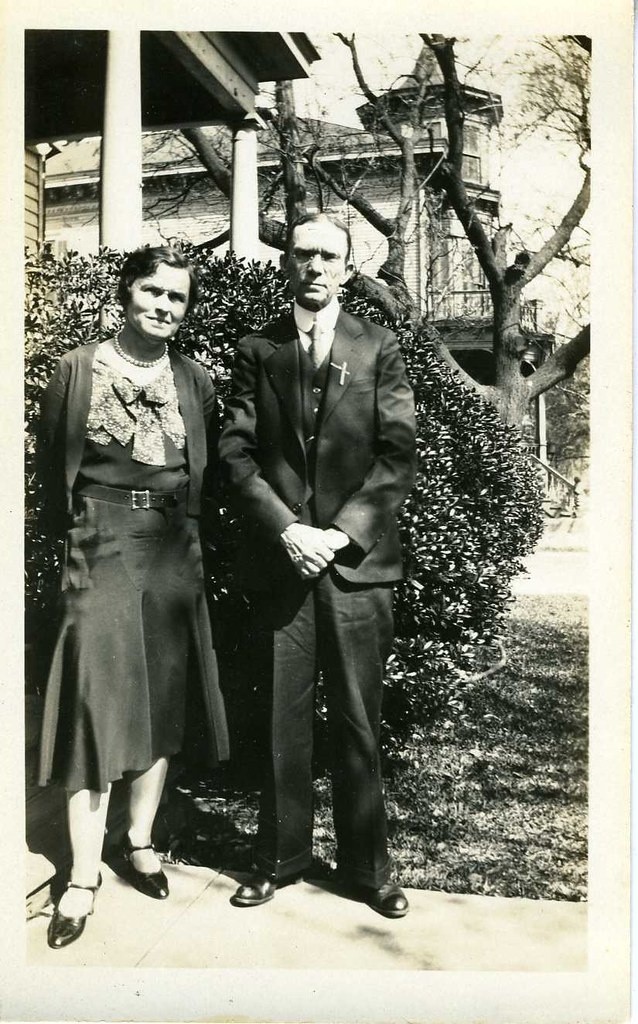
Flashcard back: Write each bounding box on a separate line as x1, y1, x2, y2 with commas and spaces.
114, 330, 169, 367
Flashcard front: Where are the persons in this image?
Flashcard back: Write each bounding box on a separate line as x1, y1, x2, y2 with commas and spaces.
213, 211, 415, 916
25, 248, 232, 948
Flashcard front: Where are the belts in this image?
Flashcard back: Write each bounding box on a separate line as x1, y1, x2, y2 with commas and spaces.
74, 482, 189, 511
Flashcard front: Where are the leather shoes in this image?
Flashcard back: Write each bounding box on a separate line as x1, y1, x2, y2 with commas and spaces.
234, 866, 304, 905
357, 879, 409, 917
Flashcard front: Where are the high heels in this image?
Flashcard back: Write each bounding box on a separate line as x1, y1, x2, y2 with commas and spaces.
47, 871, 102, 949
122, 830, 169, 899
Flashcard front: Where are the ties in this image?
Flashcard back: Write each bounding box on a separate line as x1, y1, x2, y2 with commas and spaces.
306, 323, 323, 368
